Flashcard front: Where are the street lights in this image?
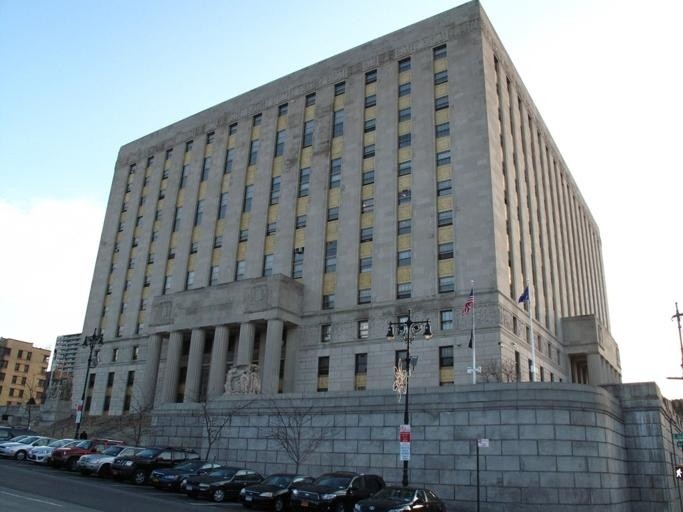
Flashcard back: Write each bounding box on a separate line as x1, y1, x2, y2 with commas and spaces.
74, 328, 103, 440
385, 309, 433, 488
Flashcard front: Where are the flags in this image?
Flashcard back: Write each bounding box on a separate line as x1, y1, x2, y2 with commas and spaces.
519, 286, 529, 303
459, 288, 474, 315
